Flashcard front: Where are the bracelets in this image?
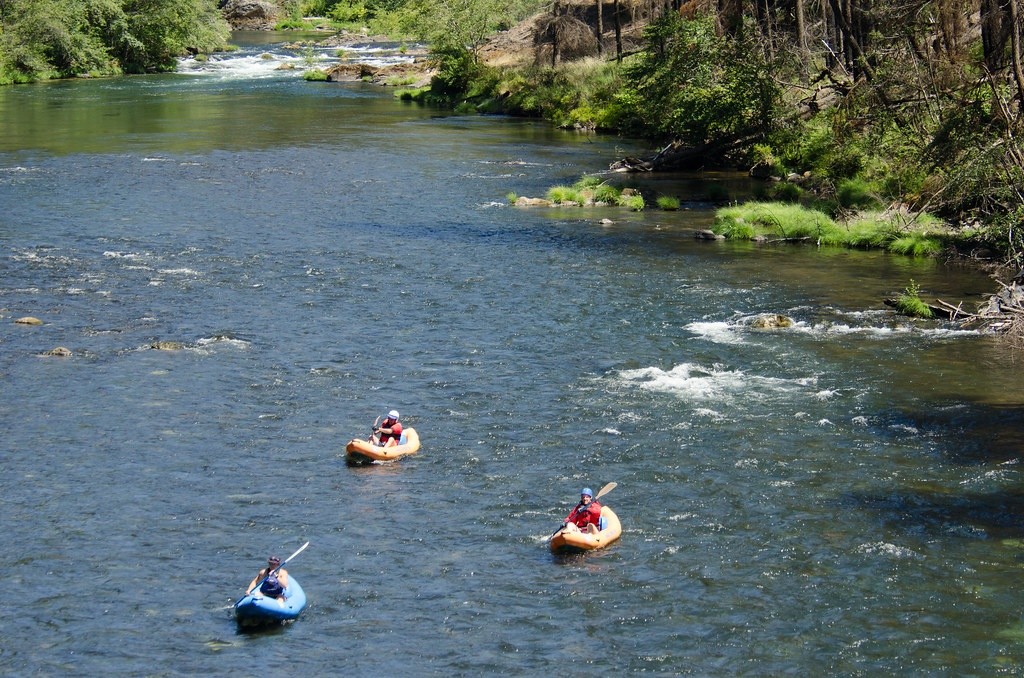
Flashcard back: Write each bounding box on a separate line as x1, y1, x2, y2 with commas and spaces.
275, 575, 278, 579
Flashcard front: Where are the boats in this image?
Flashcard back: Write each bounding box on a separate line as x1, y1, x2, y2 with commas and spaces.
550, 506, 621, 549
346, 428, 420, 461
235, 569, 306, 622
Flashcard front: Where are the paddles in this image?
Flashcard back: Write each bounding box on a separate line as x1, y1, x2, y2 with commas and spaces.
230, 541, 312, 608
542, 480, 620, 537
366, 414, 382, 445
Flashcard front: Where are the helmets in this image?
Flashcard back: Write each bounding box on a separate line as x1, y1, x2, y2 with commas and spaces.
581, 487, 593, 497
387, 410, 399, 423
268, 555, 280, 564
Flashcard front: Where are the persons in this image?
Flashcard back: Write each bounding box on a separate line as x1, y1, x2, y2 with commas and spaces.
565, 487, 601, 534
245, 555, 288, 605
367, 410, 402, 447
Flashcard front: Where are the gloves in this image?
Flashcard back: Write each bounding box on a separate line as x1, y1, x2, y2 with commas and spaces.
576, 505, 586, 513
560, 522, 566, 527
